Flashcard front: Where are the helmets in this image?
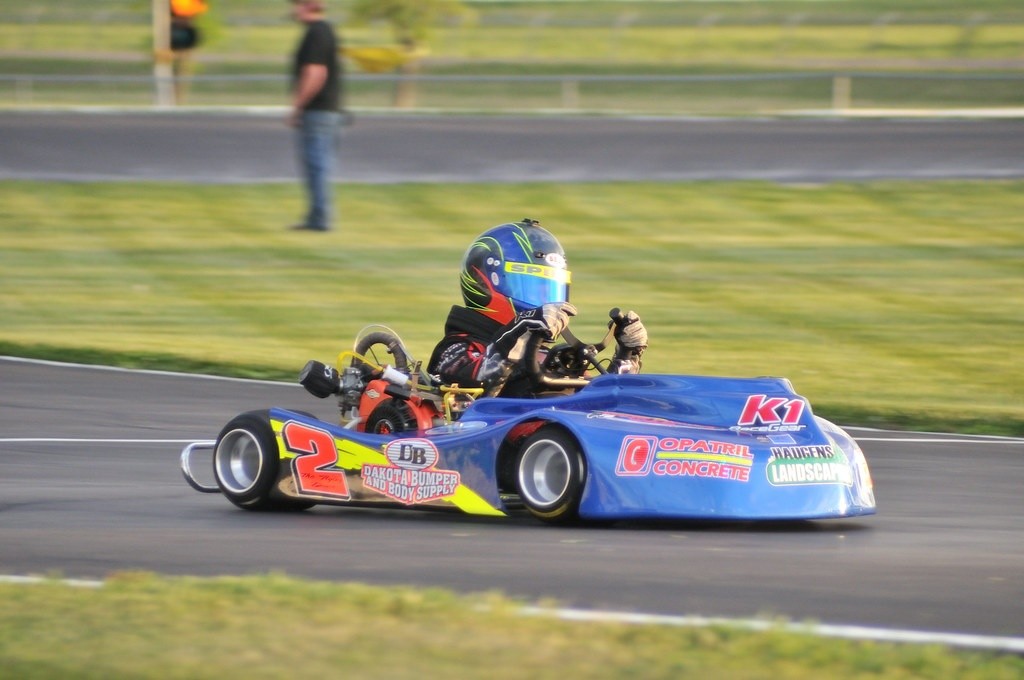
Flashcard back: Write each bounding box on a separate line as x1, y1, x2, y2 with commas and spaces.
460, 218, 571, 325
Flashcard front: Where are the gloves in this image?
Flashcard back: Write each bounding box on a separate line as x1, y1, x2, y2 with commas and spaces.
492, 302, 577, 364
607, 311, 647, 374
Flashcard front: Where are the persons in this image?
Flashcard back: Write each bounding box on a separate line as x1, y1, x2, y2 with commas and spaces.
285, 0, 343, 231
428, 219, 648, 397
